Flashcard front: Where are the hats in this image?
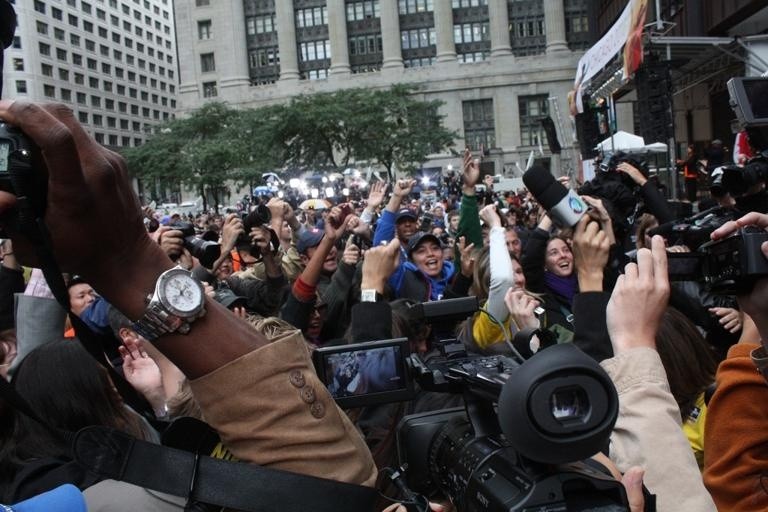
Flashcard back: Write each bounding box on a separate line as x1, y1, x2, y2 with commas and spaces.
295, 229, 325, 254
395, 207, 418, 222
405, 231, 441, 255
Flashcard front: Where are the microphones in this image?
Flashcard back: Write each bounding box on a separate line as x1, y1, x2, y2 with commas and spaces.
523, 166, 602, 233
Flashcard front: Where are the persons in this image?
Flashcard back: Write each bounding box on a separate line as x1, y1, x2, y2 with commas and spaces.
0, 93, 767, 512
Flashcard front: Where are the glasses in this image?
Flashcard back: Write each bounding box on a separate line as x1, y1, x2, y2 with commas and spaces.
308, 302, 329, 318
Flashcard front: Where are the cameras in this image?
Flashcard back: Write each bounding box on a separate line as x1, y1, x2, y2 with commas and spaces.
0, 126, 49, 268
336, 230, 362, 251
233, 204, 272, 258
168, 222, 222, 269
